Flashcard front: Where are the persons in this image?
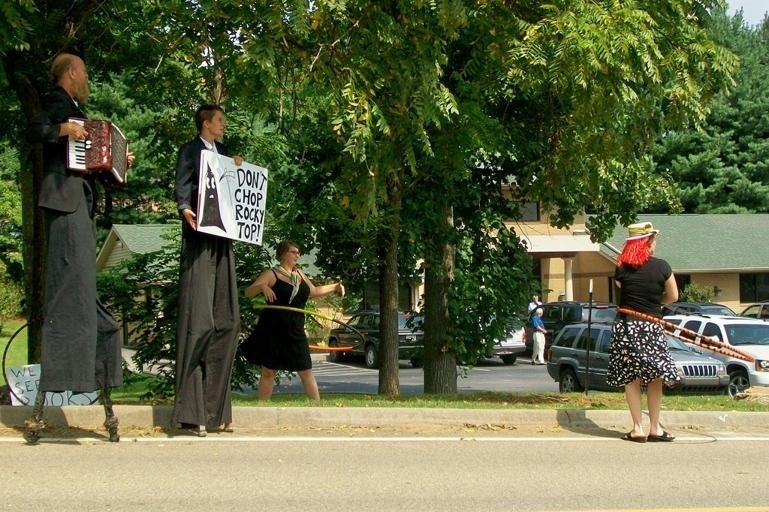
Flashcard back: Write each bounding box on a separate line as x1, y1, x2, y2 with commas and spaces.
243, 238, 347, 400
525, 291, 544, 324
19, 48, 136, 393
606, 219, 681, 443
528, 307, 550, 366
169, 100, 247, 427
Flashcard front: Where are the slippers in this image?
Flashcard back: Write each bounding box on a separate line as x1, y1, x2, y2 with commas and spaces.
620, 431, 647, 443
646, 429, 675, 442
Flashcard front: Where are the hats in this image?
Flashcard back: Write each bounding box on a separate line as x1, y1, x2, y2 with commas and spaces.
624, 221, 659, 240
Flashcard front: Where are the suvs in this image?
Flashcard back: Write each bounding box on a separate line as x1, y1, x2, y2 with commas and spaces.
660, 316, 769, 391
544, 327, 730, 400
532, 297, 617, 361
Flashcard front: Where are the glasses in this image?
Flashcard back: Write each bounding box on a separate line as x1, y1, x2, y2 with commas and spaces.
287, 250, 300, 255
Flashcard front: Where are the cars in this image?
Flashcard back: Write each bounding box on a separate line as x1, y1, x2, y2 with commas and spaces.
664, 300, 737, 325
444, 300, 531, 373
328, 309, 433, 369
